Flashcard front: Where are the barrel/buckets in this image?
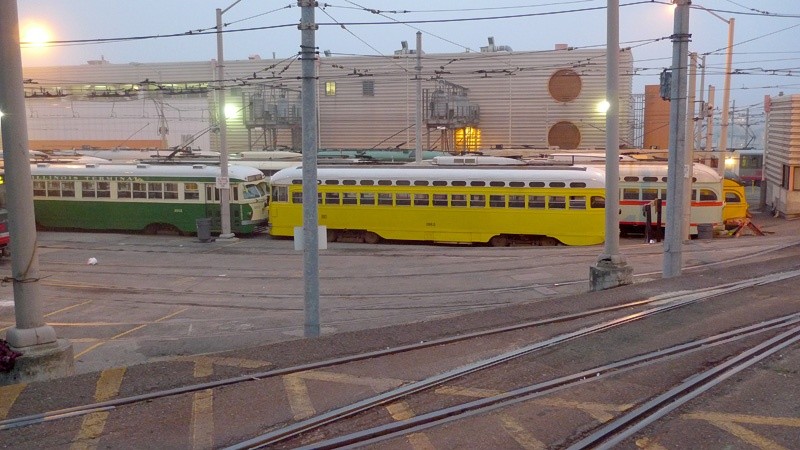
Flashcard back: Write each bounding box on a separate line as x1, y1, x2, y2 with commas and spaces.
196, 218, 211, 240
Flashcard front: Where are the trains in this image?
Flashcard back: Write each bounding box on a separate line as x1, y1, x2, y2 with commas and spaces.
268, 165, 608, 247
0, 101, 748, 235
0, 162, 270, 236
